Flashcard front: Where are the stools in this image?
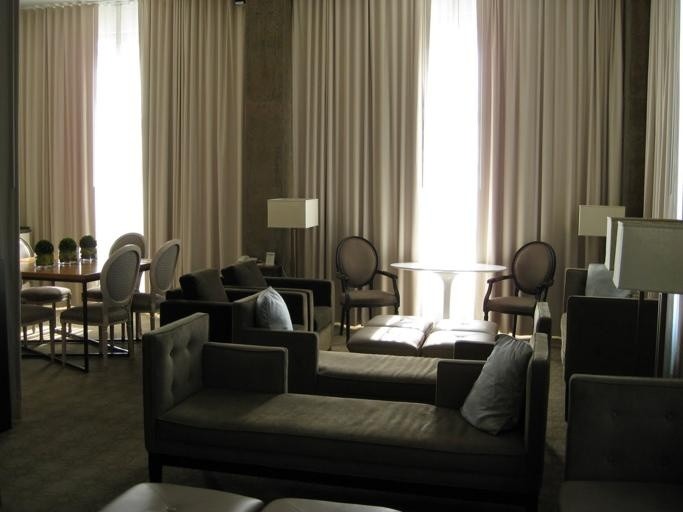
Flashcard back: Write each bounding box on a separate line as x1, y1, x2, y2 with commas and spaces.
100, 482, 397, 511
347, 314, 501, 359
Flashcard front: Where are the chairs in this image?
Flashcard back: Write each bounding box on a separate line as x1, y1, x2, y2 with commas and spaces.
335, 236, 400, 344
481, 240, 557, 337
18, 232, 183, 374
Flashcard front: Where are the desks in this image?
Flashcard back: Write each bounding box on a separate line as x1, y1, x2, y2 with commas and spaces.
389, 261, 505, 320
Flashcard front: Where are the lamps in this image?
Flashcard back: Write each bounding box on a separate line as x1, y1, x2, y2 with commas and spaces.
267, 196, 320, 278
577, 204, 626, 237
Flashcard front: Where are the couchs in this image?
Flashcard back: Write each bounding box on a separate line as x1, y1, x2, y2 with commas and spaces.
559, 266, 660, 427
141, 288, 552, 511
161, 256, 333, 350
558, 372, 681, 512
